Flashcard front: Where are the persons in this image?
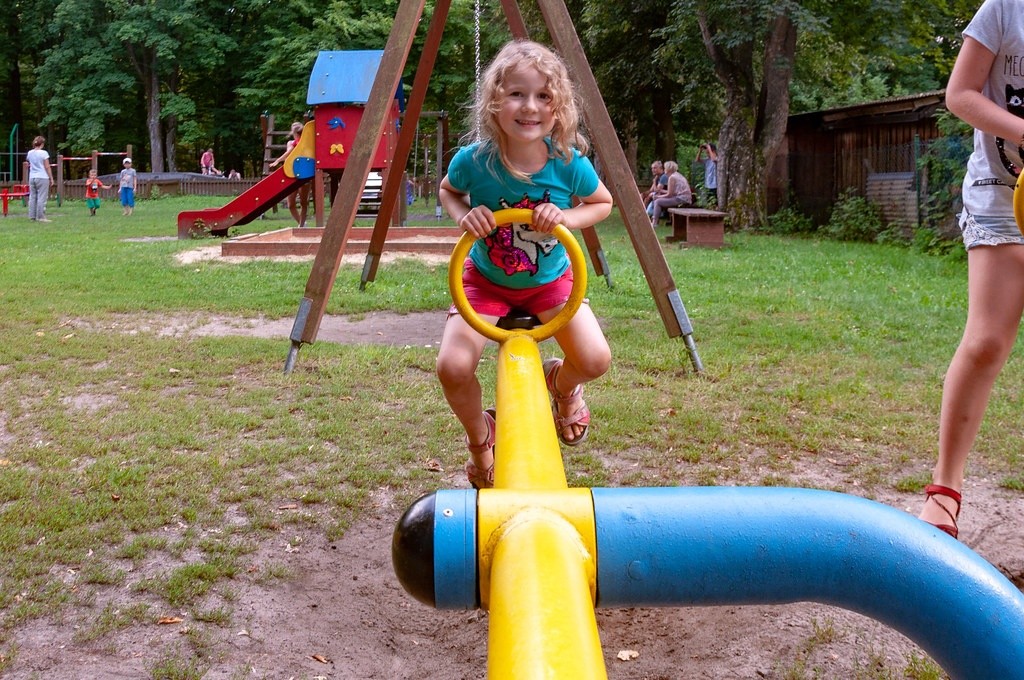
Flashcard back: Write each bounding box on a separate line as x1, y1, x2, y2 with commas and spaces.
405, 174, 416, 204
26, 136, 53, 222
228, 169, 241, 181
640, 160, 692, 226
915, 0, 1024, 539
695, 142, 717, 206
85, 169, 112, 216
435, 38, 610, 491
269, 121, 311, 229
118, 158, 137, 215
201, 148, 225, 175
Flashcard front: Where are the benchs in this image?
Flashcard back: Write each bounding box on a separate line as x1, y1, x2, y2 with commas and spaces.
665, 208, 732, 249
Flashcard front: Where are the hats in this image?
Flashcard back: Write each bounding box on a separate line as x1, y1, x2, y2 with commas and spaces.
697, 143, 717, 151
122, 157, 132, 165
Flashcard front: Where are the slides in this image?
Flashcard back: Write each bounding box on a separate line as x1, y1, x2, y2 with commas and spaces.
177, 121, 319, 239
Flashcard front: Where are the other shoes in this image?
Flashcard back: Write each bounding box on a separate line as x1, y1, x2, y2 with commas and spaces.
29, 218, 50, 223
298, 221, 307, 228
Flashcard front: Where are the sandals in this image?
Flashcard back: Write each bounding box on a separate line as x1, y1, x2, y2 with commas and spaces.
543, 358, 591, 446
464, 408, 495, 488
918, 483, 962, 540
